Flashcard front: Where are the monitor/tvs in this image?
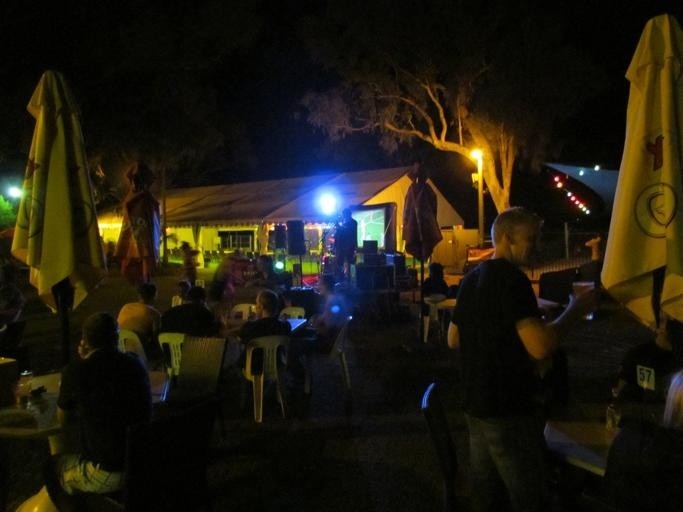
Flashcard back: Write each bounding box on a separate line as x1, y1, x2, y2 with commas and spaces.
345, 203, 397, 252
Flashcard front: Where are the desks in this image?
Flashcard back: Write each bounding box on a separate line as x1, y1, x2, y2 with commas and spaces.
0, 407, 60, 462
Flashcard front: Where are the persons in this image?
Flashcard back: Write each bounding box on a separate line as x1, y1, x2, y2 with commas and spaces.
55, 313, 150, 495
332, 208, 357, 281
662, 369, 682, 428
116, 273, 355, 389
420, 263, 448, 317
446, 206, 595, 512
180, 240, 200, 291
607, 320, 683, 403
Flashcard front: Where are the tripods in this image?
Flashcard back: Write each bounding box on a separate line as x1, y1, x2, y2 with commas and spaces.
339, 254, 356, 295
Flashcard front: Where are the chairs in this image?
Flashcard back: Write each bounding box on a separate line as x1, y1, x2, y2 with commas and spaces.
114, 298, 352, 422
418, 379, 479, 512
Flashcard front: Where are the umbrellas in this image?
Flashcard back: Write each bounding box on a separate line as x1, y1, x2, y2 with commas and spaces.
599, 12, 681, 380
10, 69, 106, 336
402, 162, 442, 322
115, 160, 160, 285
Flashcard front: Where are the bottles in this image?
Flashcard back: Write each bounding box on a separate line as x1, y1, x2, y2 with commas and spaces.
605, 399, 623, 431
19, 370, 33, 392
215, 303, 222, 323
248, 306, 255, 319
224, 303, 234, 329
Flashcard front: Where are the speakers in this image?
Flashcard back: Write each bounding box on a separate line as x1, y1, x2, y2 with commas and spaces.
275, 225, 287, 248
288, 220, 306, 254
356, 264, 393, 289
363, 241, 377, 264
386, 252, 405, 285
343, 219, 357, 254
290, 286, 314, 323
397, 269, 417, 289
